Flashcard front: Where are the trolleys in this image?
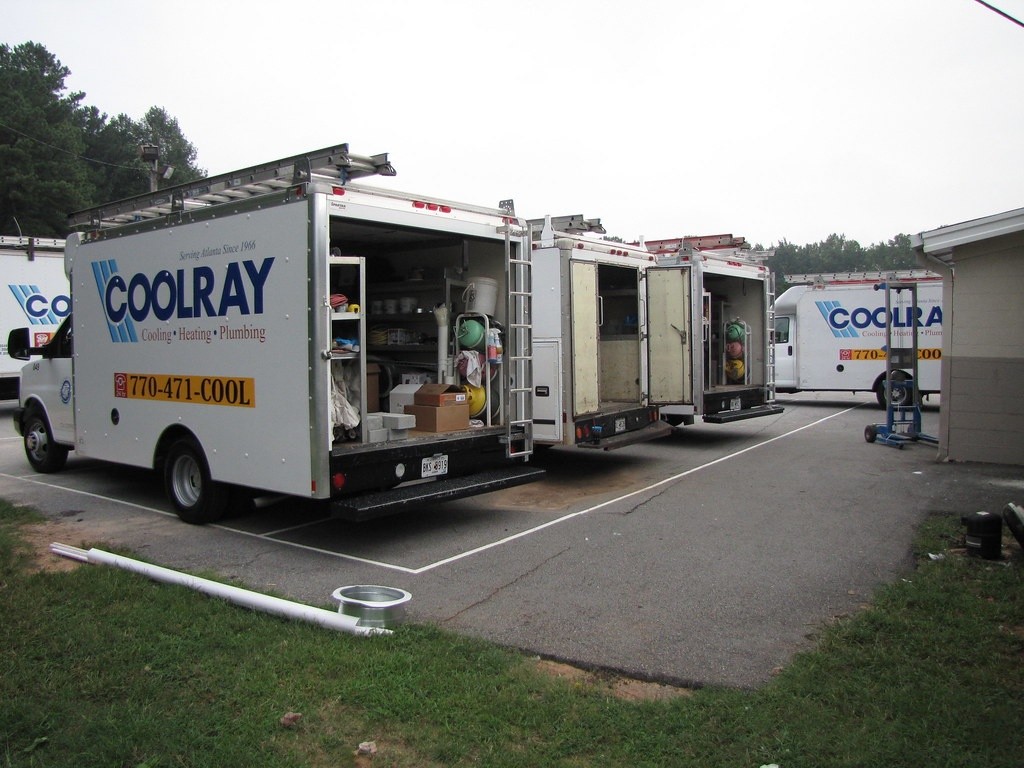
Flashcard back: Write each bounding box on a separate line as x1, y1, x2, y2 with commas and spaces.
863, 277, 941, 449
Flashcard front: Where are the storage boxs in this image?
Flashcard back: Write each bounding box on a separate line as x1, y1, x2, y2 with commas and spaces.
414, 384, 467, 407
402, 371, 437, 384
390, 384, 423, 413
388, 329, 406, 345
404, 405, 470, 432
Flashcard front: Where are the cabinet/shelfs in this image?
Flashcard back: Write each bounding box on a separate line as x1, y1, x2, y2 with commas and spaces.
705, 301, 731, 385
330, 256, 368, 444
703, 291, 712, 391
366, 277, 467, 384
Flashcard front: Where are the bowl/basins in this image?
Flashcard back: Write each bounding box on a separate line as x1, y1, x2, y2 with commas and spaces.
332, 585, 412, 628
412, 307, 423, 313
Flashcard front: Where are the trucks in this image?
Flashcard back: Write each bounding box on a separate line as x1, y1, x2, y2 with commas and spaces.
0, 234, 71, 401
517, 215, 787, 454
8, 139, 539, 527
768, 268, 943, 412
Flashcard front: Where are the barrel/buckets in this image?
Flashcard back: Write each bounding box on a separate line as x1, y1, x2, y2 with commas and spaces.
458, 353, 497, 382
459, 320, 485, 353
384, 299, 398, 314
400, 297, 418, 313
462, 276, 499, 316
461, 383, 499, 419
